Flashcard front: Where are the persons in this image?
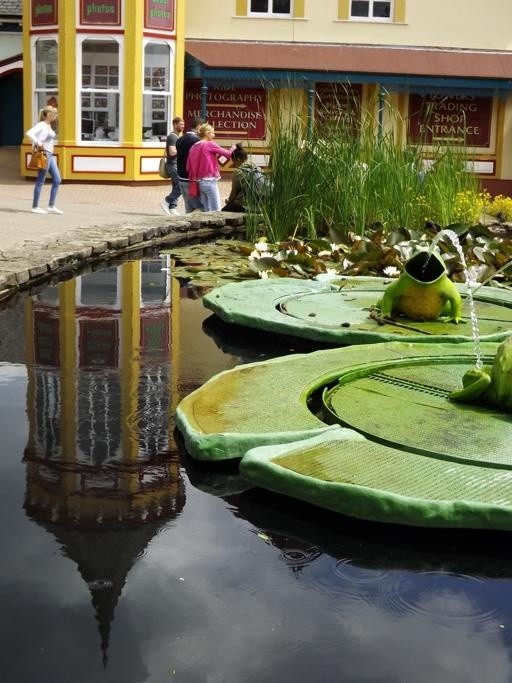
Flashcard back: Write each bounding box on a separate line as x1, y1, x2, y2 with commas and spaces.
176, 115, 205, 212
26, 106, 64, 216
186, 123, 237, 214
160, 117, 185, 216
221, 142, 262, 213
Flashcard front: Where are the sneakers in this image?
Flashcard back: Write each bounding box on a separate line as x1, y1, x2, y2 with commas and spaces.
47, 205, 62, 215
160, 198, 177, 215
32, 206, 46, 214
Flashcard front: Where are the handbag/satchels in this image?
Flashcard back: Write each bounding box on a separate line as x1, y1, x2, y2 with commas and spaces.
159, 158, 168, 178
189, 181, 198, 195
29, 151, 47, 170
